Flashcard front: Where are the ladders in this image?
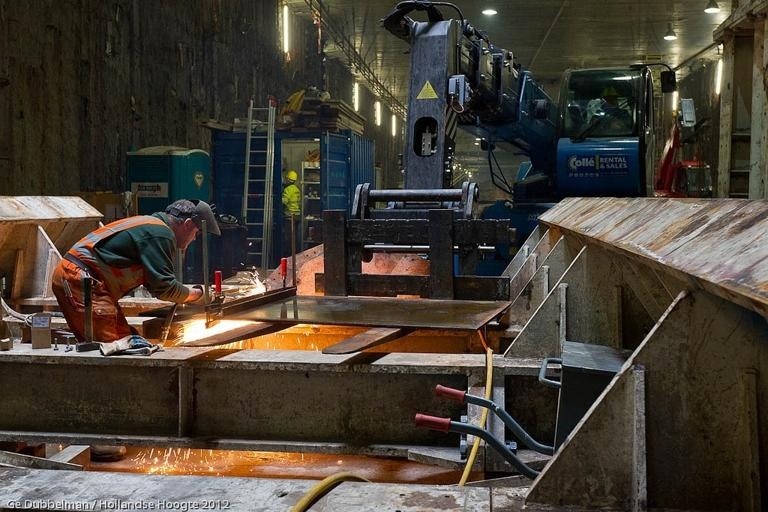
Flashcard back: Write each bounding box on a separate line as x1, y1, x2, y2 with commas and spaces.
240, 99, 276, 271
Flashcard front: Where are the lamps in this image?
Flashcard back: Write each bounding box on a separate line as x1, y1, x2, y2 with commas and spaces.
704, 0, 720, 13
664, 22, 677, 40
481, 0, 498, 15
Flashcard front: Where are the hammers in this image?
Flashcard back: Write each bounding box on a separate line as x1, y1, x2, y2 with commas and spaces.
76, 278, 100, 352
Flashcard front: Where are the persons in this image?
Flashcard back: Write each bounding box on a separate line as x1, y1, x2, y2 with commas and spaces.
594, 87, 633, 130
281, 170, 301, 217
50, 197, 222, 348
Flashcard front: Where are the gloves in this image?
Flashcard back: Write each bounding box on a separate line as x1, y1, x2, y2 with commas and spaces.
100, 335, 152, 356
186, 285, 212, 304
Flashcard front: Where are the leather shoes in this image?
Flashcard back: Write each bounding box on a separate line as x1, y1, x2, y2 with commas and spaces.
90, 445, 126, 462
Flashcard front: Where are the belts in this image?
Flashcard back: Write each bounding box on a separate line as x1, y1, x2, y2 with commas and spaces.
64, 253, 98, 279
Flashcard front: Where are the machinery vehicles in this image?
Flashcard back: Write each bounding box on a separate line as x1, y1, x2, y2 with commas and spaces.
349, 0, 678, 275
655, 98, 717, 197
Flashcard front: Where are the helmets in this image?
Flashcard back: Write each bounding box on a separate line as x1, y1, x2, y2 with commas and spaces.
286, 170, 297, 180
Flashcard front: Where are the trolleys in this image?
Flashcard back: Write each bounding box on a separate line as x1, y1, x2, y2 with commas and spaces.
411, 338, 633, 479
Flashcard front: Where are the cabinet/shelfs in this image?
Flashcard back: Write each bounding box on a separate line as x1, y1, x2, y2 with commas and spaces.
300, 161, 325, 250
